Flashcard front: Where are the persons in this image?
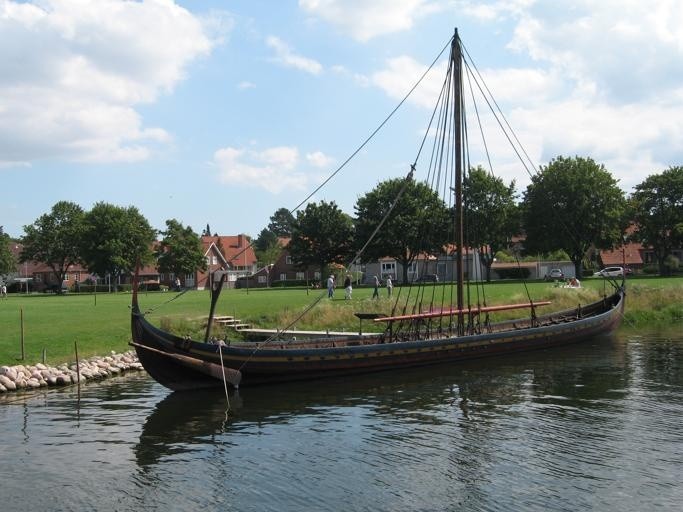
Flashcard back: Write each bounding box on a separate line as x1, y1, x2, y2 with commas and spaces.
2, 285, 7, 299
176, 277, 181, 291
387, 275, 392, 298
75, 279, 80, 294
555, 273, 581, 288
344, 274, 352, 300
372, 276, 381, 299
328, 275, 335, 298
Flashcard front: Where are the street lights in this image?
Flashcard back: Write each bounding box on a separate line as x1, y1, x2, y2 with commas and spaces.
16, 244, 29, 294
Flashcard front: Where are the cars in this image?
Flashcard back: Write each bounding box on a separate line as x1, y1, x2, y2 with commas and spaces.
415, 274, 439, 282
549, 268, 561, 278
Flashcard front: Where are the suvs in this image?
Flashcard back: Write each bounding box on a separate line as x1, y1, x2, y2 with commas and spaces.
592, 266, 623, 279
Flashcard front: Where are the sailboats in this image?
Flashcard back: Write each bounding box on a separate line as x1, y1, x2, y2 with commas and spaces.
127, 24, 624, 394
